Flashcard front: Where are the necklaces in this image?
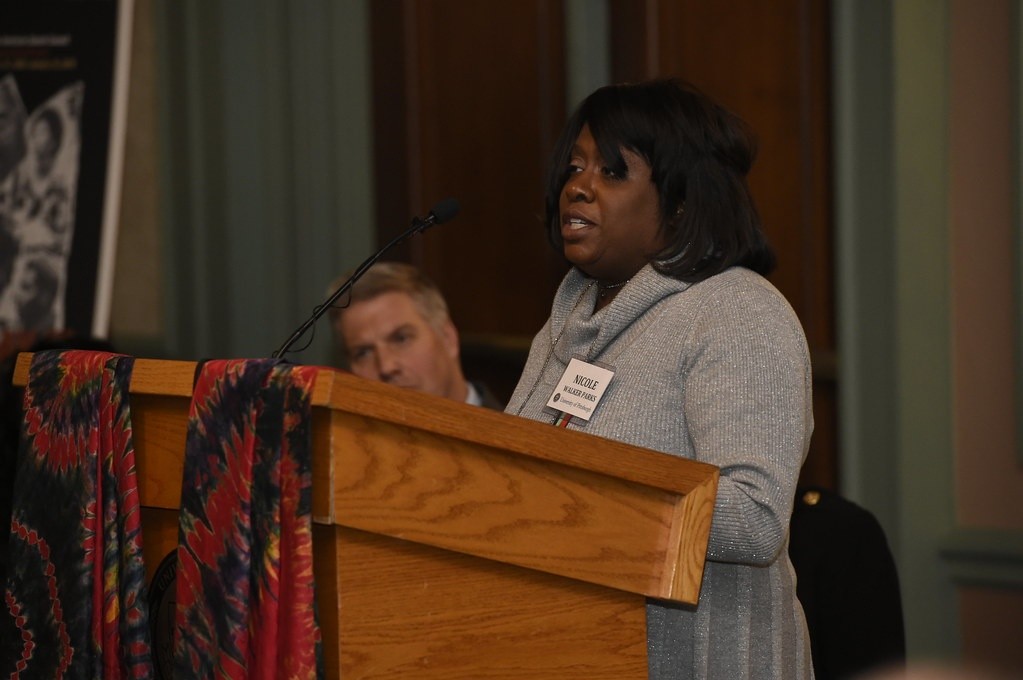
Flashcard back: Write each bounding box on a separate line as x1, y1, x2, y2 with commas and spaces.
594, 278, 629, 298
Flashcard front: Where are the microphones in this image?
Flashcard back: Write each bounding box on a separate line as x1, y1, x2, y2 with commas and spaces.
412, 215, 432, 234
272, 198, 461, 361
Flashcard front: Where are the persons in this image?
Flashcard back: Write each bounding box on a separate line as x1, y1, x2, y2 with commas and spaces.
788, 486, 906, 680
503, 79, 819, 680
323, 260, 506, 413
